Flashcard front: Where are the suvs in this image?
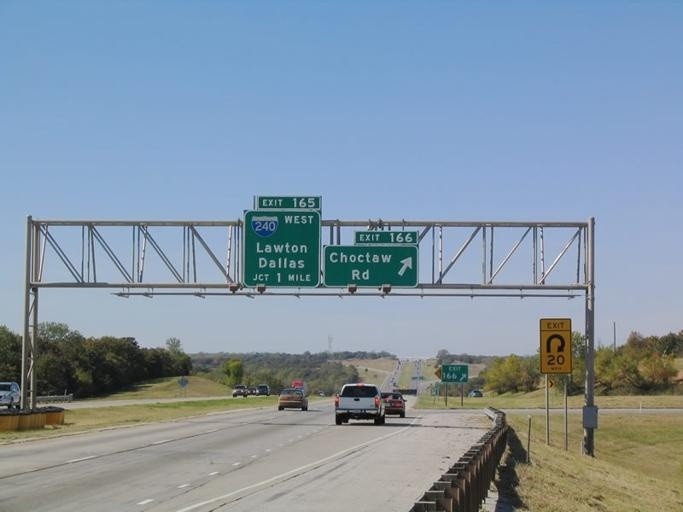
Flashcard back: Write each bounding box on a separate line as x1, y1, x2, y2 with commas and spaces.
231, 383, 249, 399
255, 383, 271, 396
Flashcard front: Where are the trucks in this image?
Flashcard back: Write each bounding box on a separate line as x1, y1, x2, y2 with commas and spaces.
290, 380, 305, 396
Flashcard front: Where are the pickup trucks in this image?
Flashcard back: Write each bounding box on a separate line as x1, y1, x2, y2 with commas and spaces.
332, 383, 386, 424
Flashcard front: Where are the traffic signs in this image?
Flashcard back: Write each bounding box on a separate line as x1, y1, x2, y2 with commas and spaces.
257, 196, 322, 212
352, 230, 418, 246
243, 209, 321, 289
322, 244, 419, 290
440, 363, 468, 384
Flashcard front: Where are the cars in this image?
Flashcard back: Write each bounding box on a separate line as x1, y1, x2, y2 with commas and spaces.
0, 380, 22, 410
314, 390, 331, 398
468, 389, 482, 399
277, 387, 308, 411
247, 387, 256, 395
380, 391, 407, 417
